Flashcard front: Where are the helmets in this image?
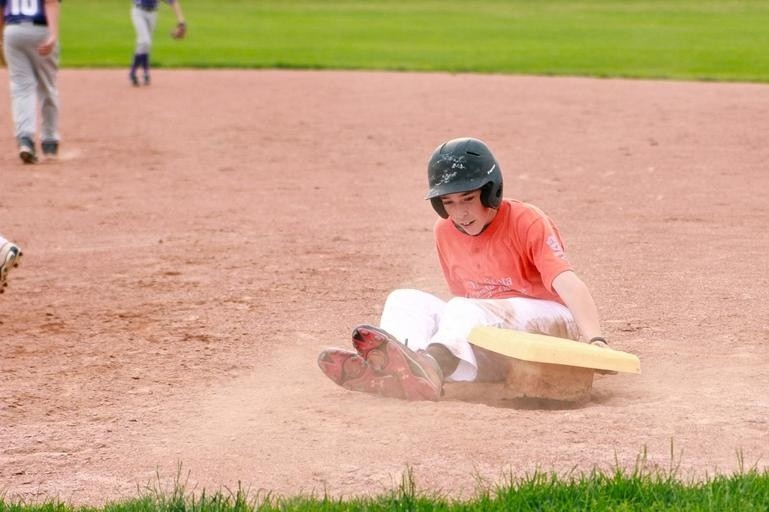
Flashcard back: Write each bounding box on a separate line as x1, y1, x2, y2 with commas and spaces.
425, 137, 504, 221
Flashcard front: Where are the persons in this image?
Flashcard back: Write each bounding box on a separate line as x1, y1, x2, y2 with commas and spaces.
129, 0, 185, 86
0, 0, 60, 162
318, 137, 617, 402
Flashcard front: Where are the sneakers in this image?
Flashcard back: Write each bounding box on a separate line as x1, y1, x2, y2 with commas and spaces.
1, 242, 23, 294
318, 325, 444, 403
19, 138, 60, 164
130, 69, 150, 87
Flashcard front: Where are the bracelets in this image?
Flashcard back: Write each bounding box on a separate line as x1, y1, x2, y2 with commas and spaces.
589, 337, 607, 344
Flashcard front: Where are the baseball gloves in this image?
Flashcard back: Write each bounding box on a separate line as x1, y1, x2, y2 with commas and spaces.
170, 22, 187, 40
